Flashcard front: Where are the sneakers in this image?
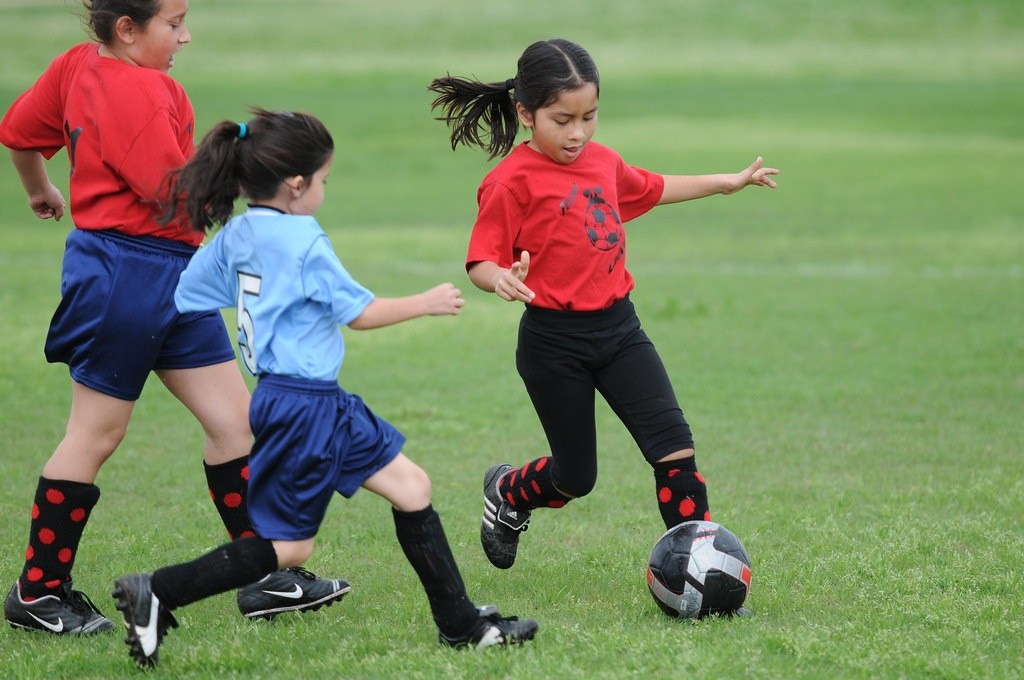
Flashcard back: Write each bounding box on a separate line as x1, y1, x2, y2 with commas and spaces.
481, 464, 531, 569
113, 574, 179, 674
439, 605, 539, 652
5, 577, 117, 637
237, 565, 351, 623
736, 605, 752, 618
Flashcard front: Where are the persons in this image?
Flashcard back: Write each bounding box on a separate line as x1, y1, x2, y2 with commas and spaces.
425, 38, 782, 620
111, 104, 540, 674
0, 0, 351, 638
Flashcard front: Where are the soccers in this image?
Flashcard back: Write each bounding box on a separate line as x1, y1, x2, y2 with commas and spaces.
646, 520, 753, 625
